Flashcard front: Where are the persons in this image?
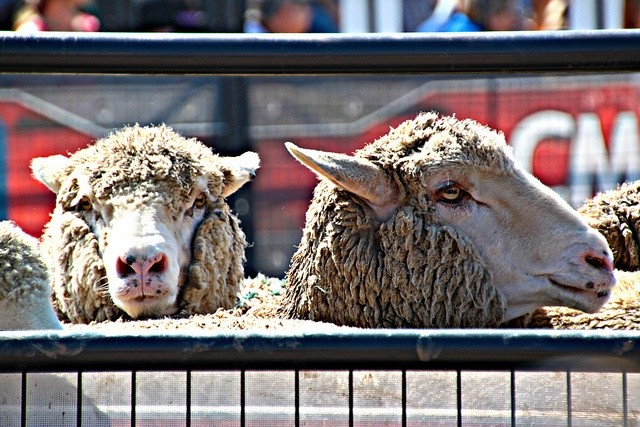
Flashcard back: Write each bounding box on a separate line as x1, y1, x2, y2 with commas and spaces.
248, 0, 314, 34
416, 0, 541, 31
14, 0, 101, 32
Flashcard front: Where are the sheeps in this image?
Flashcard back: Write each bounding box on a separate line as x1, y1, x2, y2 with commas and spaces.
275, 109, 640, 331
30, 122, 275, 324
